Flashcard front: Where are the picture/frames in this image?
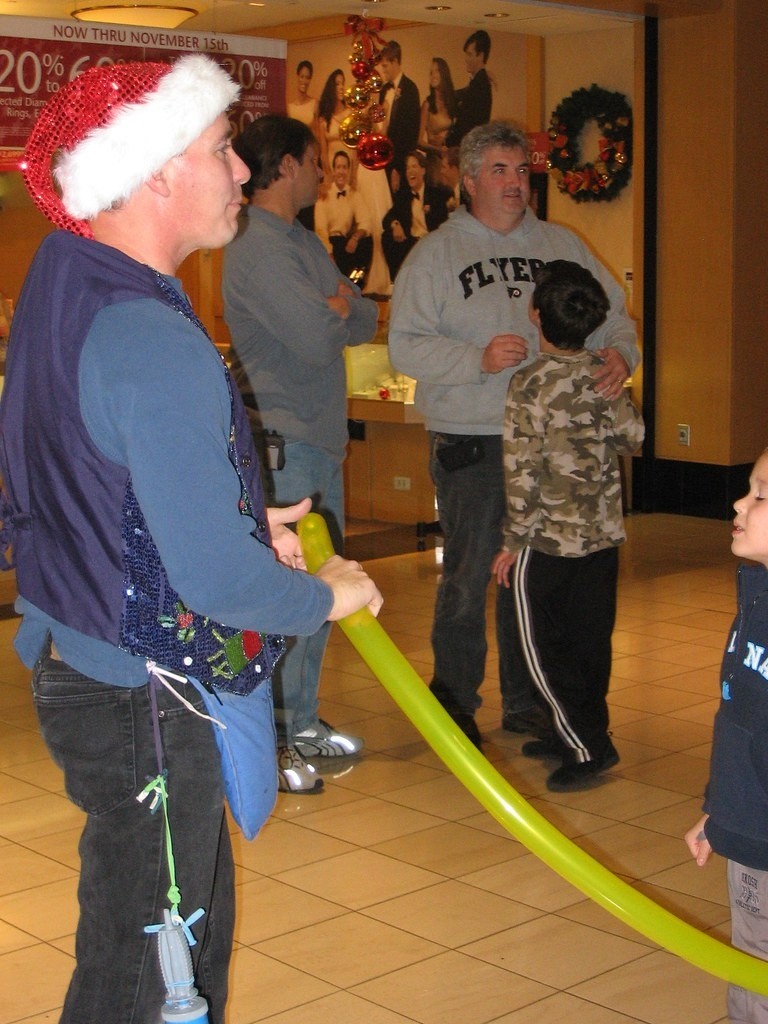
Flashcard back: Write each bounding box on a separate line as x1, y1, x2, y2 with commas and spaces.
232, 15, 543, 318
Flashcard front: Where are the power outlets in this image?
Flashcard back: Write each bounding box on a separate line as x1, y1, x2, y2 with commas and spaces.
677, 423, 691, 446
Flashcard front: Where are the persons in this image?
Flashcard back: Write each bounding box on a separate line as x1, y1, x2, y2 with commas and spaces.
0, 49, 385, 1024
492, 259, 645, 792
389, 120, 641, 750
218, 112, 380, 795
684, 448, 768, 1024
287, 27, 491, 294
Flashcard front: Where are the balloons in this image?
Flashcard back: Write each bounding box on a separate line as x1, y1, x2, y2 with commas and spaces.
295, 514, 768, 997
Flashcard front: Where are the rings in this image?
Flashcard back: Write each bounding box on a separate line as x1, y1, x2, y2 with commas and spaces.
617, 379, 623, 384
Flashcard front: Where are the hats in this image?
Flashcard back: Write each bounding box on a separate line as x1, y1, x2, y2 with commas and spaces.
20, 52, 242, 241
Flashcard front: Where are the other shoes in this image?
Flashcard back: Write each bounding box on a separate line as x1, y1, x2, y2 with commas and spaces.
546, 730, 621, 792
454, 716, 482, 746
503, 706, 558, 741
521, 738, 566, 756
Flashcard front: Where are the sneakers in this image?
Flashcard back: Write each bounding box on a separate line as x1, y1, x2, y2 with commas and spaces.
274, 718, 364, 757
278, 740, 324, 794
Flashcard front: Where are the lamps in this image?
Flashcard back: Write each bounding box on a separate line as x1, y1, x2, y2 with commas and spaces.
70, 0, 204, 29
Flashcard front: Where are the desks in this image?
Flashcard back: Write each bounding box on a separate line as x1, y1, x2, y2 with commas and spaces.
344, 399, 441, 551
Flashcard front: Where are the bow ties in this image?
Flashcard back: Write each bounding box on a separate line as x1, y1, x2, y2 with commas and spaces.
411, 192, 420, 200
386, 80, 394, 89
337, 191, 346, 198
